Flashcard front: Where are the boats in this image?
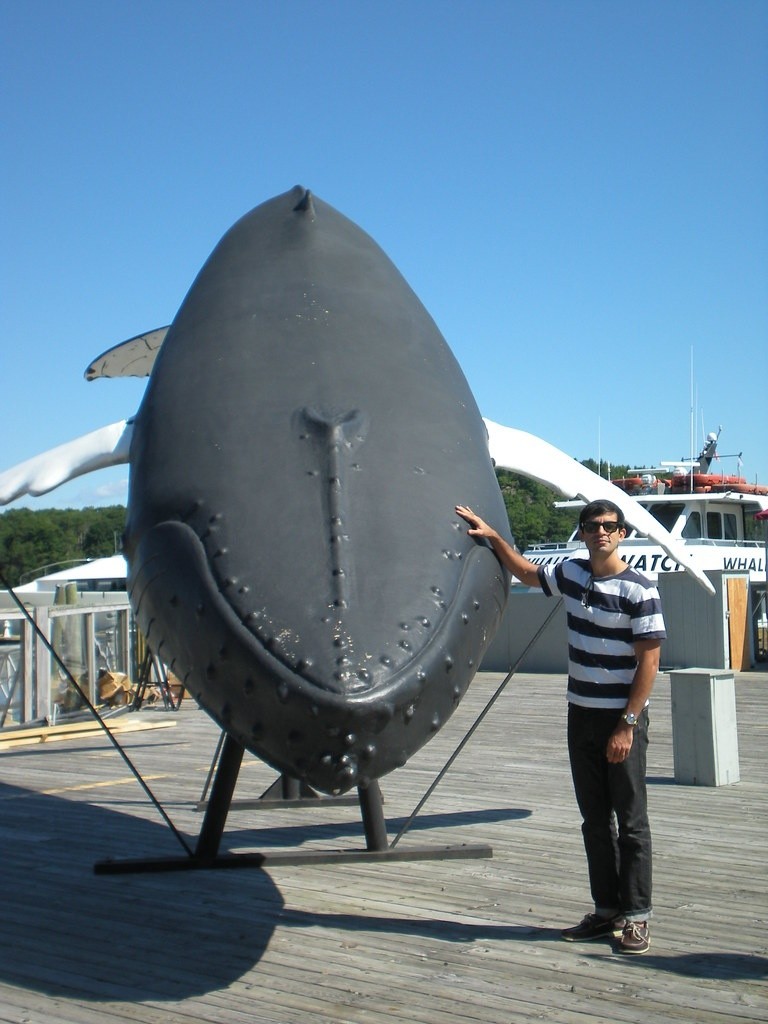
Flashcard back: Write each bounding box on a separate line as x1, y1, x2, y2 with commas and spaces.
0, 529, 137, 654
521, 345, 768, 629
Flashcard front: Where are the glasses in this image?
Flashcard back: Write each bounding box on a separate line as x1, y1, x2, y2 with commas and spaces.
582, 521, 621, 534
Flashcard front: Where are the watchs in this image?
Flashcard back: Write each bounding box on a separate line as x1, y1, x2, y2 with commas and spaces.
621, 714, 639, 727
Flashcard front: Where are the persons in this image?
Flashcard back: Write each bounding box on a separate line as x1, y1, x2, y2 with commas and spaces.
456, 499, 668, 954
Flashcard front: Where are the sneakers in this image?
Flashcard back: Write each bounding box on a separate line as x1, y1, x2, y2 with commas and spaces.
561, 910, 625, 941
618, 920, 649, 954
